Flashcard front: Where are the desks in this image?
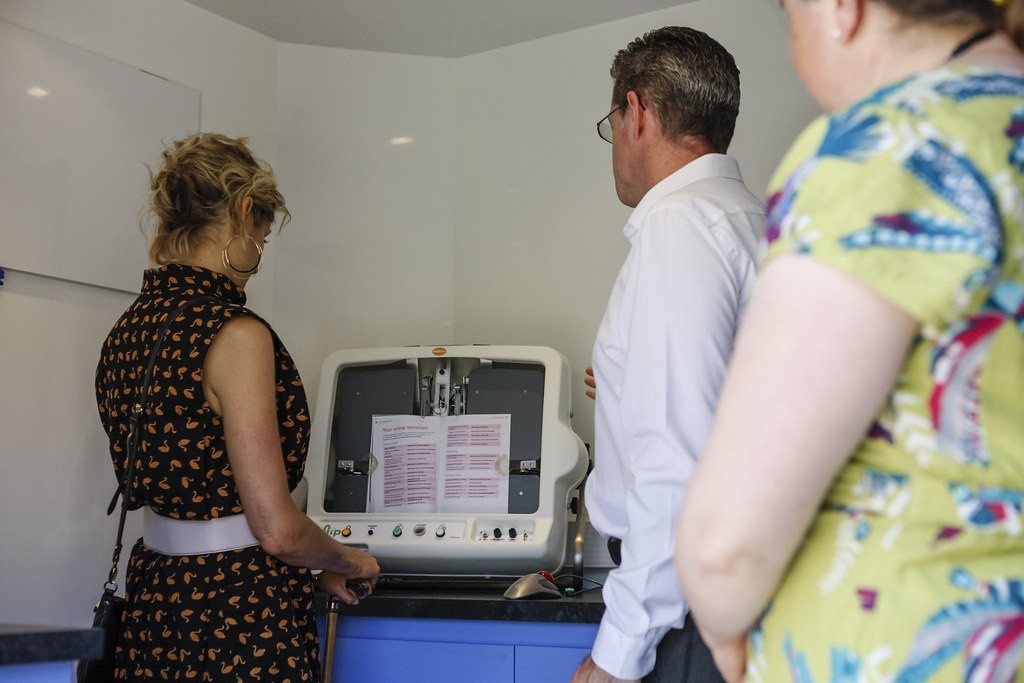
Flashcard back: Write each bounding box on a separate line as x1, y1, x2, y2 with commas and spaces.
316, 569, 610, 683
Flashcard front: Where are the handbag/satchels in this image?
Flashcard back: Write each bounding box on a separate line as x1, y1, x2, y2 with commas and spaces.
76, 580, 125, 683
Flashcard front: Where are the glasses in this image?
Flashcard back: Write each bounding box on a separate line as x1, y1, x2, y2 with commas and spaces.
597, 97, 646, 145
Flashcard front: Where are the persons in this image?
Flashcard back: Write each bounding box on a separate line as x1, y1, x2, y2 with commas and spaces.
571, 25, 765, 683
95, 133, 379, 683
675, 0, 1024, 683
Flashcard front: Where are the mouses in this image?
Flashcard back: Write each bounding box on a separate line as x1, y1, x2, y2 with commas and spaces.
503, 571, 562, 600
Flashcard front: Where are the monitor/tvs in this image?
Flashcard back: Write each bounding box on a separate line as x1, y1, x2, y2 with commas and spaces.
305, 344, 589, 589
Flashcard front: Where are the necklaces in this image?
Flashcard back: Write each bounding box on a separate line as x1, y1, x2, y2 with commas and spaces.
948, 25, 994, 66
